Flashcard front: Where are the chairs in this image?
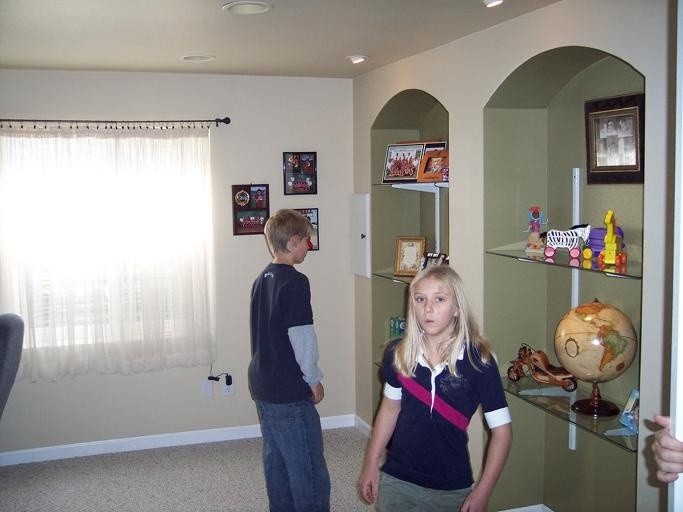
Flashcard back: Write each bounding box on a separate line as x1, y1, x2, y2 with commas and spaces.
0, 309, 26, 436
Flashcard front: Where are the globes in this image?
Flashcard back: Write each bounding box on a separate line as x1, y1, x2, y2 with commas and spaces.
554, 297, 638, 417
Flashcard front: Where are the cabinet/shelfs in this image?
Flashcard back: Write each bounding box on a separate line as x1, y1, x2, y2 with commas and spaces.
368, 176, 450, 390
482, 233, 644, 462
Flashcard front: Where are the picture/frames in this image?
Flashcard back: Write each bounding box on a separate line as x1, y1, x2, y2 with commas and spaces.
394, 236, 426, 276
285, 207, 321, 252
231, 183, 270, 237
381, 141, 447, 184
583, 90, 645, 185
282, 151, 318, 195
416, 149, 449, 182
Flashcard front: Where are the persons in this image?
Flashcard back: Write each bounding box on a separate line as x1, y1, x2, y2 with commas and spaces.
650, 414, 683, 483
598, 119, 633, 164
430, 158, 442, 171
389, 153, 414, 177
248, 209, 331, 512
239, 189, 263, 229
359, 267, 513, 512
289, 154, 311, 193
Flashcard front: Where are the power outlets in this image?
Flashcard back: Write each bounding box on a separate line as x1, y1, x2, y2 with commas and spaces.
222, 377, 234, 395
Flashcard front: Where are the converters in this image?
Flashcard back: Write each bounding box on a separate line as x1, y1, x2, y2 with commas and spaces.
226, 375, 232, 385
